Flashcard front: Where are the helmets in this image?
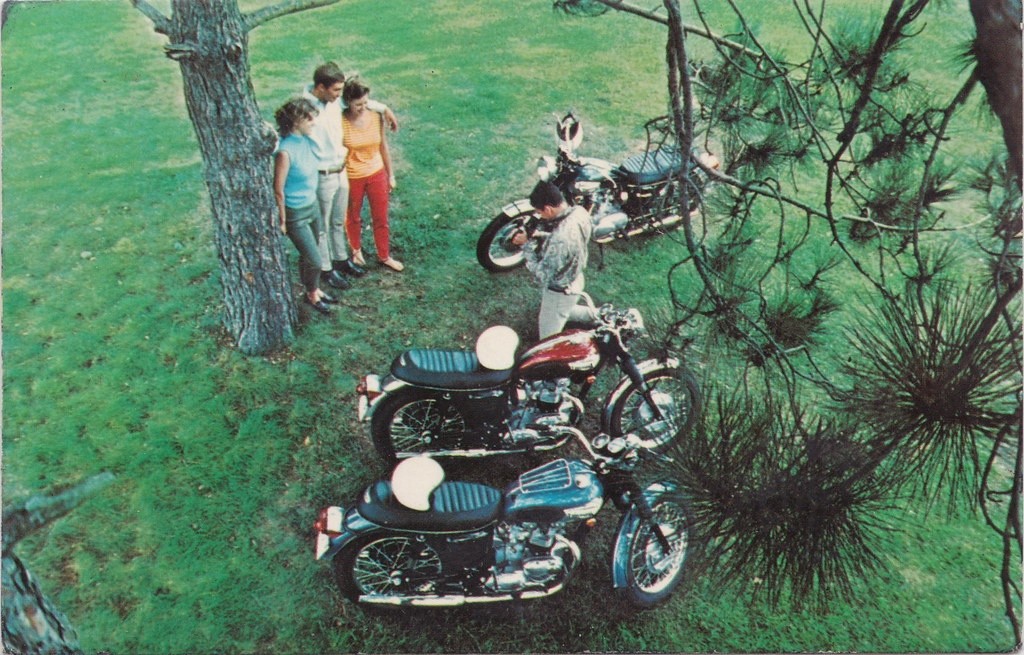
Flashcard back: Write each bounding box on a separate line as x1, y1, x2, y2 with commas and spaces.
476, 326, 520, 370
555, 114, 584, 150
390, 456, 446, 510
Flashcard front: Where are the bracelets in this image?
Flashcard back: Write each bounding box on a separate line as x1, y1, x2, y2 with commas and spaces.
388, 175, 395, 182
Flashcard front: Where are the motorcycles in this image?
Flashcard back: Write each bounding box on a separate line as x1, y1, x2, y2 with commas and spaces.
354, 282, 701, 469
476, 108, 720, 274
316, 425, 696, 614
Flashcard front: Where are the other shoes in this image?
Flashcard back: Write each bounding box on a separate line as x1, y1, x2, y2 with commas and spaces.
306, 292, 332, 314
353, 248, 365, 267
382, 257, 404, 271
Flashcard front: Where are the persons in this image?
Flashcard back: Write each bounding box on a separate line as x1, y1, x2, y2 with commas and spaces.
513, 181, 613, 340
264, 61, 404, 289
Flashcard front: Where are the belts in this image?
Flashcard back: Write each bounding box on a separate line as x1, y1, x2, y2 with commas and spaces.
318, 161, 346, 175
548, 286, 568, 293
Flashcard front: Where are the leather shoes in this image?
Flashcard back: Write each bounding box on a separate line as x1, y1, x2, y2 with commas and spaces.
333, 258, 367, 277
321, 269, 349, 289
